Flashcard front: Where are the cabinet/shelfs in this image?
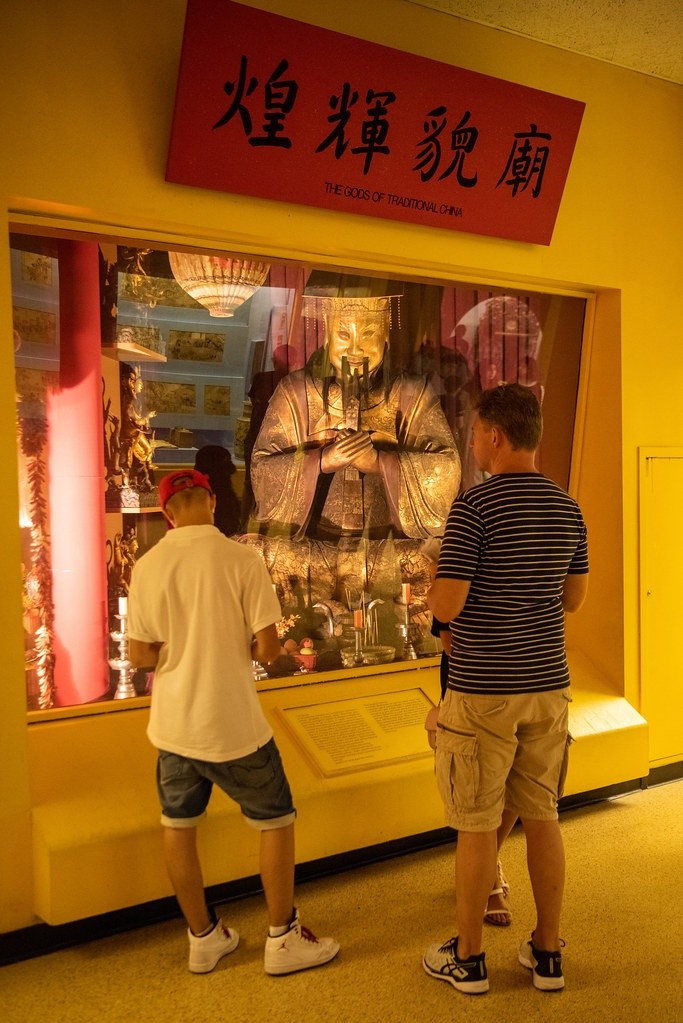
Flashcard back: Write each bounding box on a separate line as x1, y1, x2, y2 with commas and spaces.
100, 341, 168, 513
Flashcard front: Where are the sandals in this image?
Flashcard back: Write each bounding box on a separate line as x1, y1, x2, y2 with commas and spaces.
484, 859, 515, 926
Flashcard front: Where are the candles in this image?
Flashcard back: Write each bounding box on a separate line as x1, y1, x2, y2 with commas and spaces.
118, 596, 127, 616
402, 583, 411, 604
353, 610, 362, 629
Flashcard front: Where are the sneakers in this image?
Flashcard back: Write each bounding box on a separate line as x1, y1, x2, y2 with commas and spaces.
422, 936, 491, 993
263, 905, 341, 974
519, 930, 566, 990
187, 908, 241, 974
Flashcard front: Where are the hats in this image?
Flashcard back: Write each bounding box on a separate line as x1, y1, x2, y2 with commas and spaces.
158, 468, 213, 507
193, 446, 237, 474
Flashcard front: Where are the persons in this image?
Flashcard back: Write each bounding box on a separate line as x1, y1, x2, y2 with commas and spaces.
425, 382, 590, 997
126, 468, 340, 976
232, 280, 462, 674
